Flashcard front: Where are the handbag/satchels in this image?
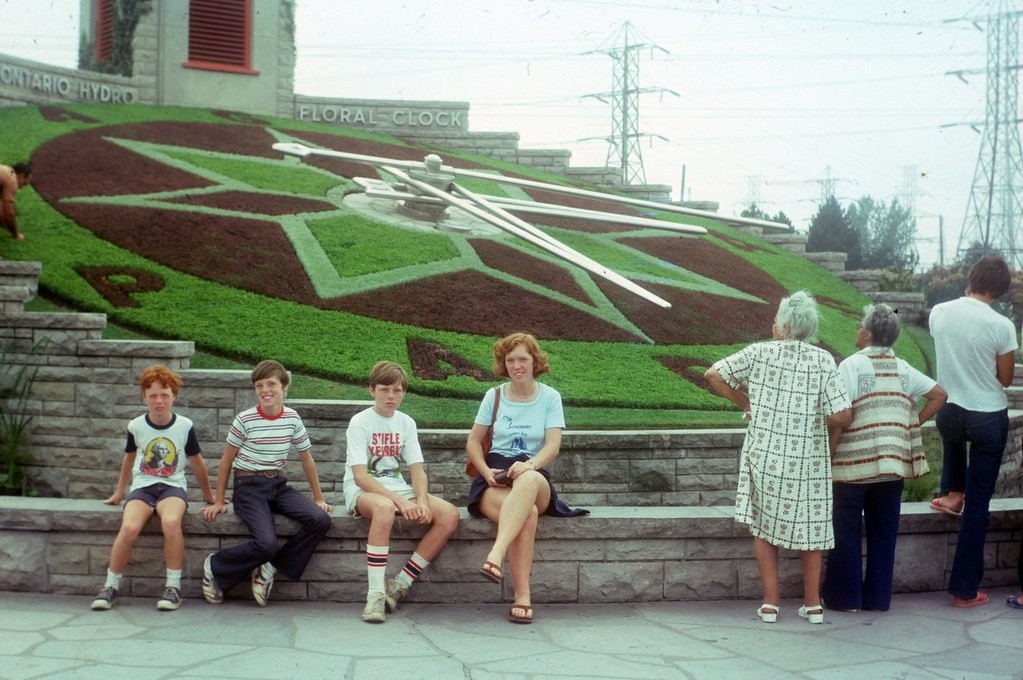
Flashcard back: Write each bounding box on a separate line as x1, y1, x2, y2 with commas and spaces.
464, 386, 500, 479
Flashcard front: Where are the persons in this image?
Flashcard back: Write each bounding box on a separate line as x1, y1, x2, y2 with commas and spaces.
343, 361, 460, 624
200, 359, 331, 605
821, 304, 947, 615
91, 366, 229, 610
1006, 532, 1023, 609
928, 256, 1017, 607
0, 162, 31, 240
703, 291, 852, 624
466, 333, 565, 624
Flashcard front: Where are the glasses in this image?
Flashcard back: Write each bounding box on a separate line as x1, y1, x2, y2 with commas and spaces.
854, 321, 864, 329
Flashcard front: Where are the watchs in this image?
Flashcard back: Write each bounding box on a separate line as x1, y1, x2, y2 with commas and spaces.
527, 459, 536, 470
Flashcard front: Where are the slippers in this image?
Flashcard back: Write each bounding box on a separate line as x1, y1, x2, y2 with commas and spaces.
929, 497, 962, 517
953, 591, 989, 607
508, 604, 534, 624
478, 560, 503, 583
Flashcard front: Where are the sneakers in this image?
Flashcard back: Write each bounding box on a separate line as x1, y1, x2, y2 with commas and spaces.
201, 553, 225, 604
156, 584, 182, 609
90, 585, 119, 609
363, 589, 386, 623
251, 563, 274, 608
383, 576, 410, 613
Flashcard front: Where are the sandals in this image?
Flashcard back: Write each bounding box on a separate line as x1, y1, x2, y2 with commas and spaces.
1005, 595, 1023, 608
756, 604, 779, 623
797, 603, 825, 624
